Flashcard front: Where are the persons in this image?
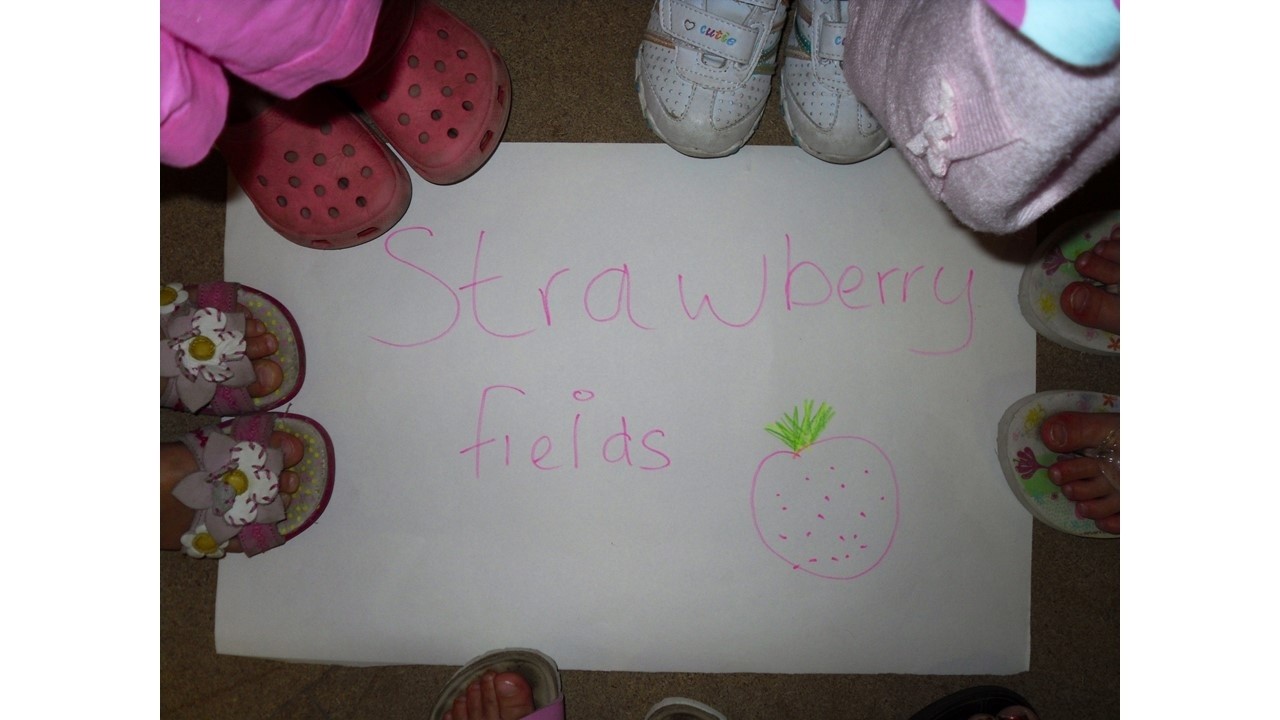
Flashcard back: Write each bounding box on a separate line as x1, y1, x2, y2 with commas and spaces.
429, 647, 731, 720
996, 192, 1120, 539
160, 280, 335, 559
905, 685, 1039, 720
160, 0, 512, 251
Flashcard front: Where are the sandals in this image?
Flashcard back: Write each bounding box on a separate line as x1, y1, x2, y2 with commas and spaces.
160, 283, 308, 417
997, 390, 1119, 540
648, 697, 723, 719
429, 647, 563, 719
1014, 217, 1120, 358
341, 0, 511, 186
222, 79, 417, 250
160, 411, 335, 558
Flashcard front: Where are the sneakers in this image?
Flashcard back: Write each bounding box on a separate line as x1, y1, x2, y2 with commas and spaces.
780, 1, 894, 163
633, 1, 789, 157
